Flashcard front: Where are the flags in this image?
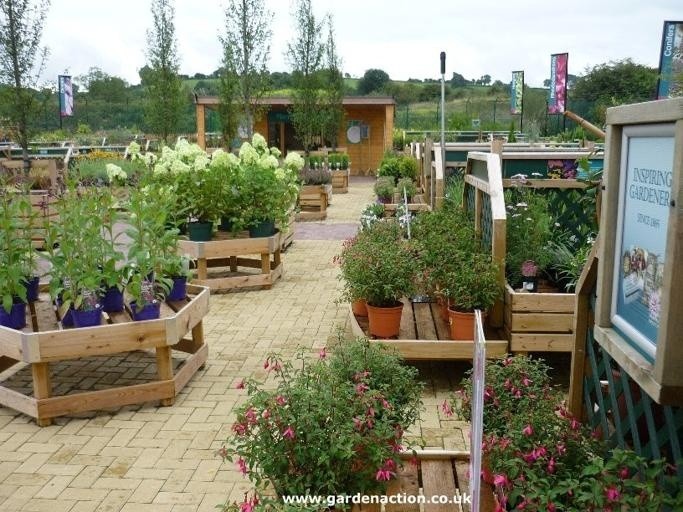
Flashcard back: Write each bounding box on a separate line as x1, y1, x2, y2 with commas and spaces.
549, 53, 567, 114
656, 21, 682, 97
510, 70, 522, 114
59, 74, 73, 117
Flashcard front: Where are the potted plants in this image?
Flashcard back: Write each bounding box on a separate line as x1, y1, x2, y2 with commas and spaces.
370, 150, 420, 203
0, 168, 186, 330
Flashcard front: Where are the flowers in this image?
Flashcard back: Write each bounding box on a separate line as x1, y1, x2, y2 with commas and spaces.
333, 208, 490, 341
103, 131, 351, 239
209, 300, 682, 511
503, 166, 596, 294
